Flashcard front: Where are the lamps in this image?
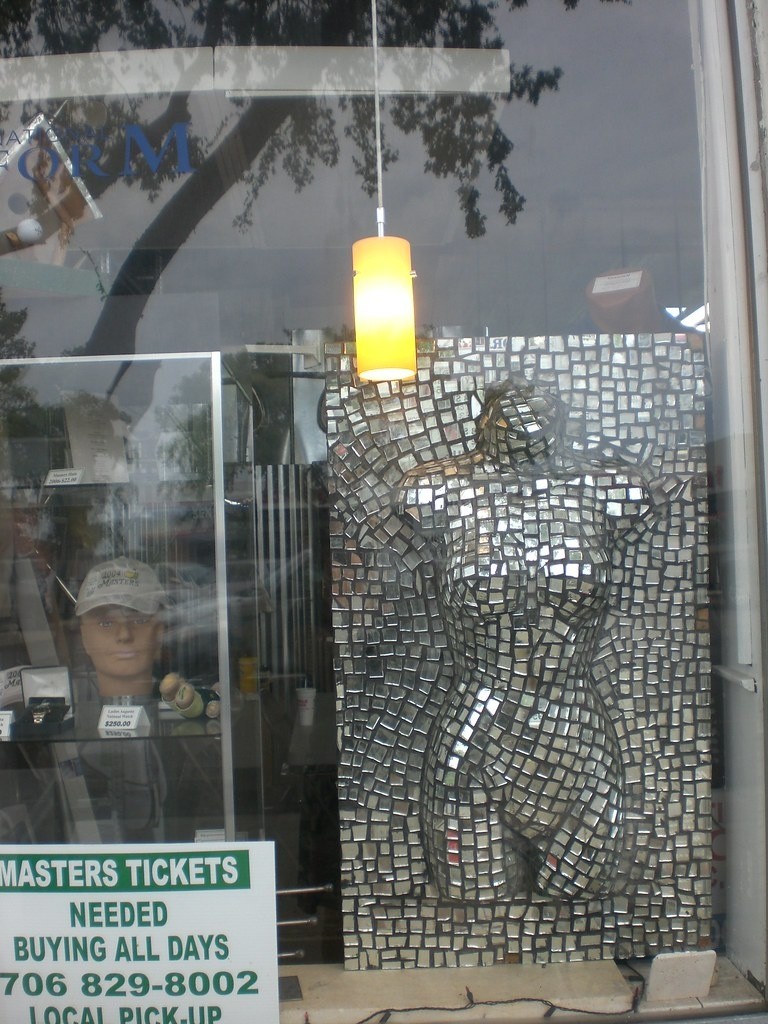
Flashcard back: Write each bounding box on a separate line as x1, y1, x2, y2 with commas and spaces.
351, 0, 419, 384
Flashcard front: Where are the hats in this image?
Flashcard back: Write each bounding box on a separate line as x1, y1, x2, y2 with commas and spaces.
74, 556, 173, 617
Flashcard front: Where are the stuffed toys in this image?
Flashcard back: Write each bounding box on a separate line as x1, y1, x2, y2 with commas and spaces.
159, 673, 243, 718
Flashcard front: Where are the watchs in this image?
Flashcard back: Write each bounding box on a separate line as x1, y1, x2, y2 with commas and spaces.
31, 702, 52, 724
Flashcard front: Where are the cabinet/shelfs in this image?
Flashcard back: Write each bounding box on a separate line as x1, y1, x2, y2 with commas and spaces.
1, 346, 265, 846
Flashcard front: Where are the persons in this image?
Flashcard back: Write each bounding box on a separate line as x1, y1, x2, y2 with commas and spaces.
198, 548, 272, 675
76, 558, 166, 703
570, 268, 699, 333
397, 384, 649, 901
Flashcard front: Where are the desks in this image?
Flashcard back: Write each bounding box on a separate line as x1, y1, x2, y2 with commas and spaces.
285, 691, 338, 836
